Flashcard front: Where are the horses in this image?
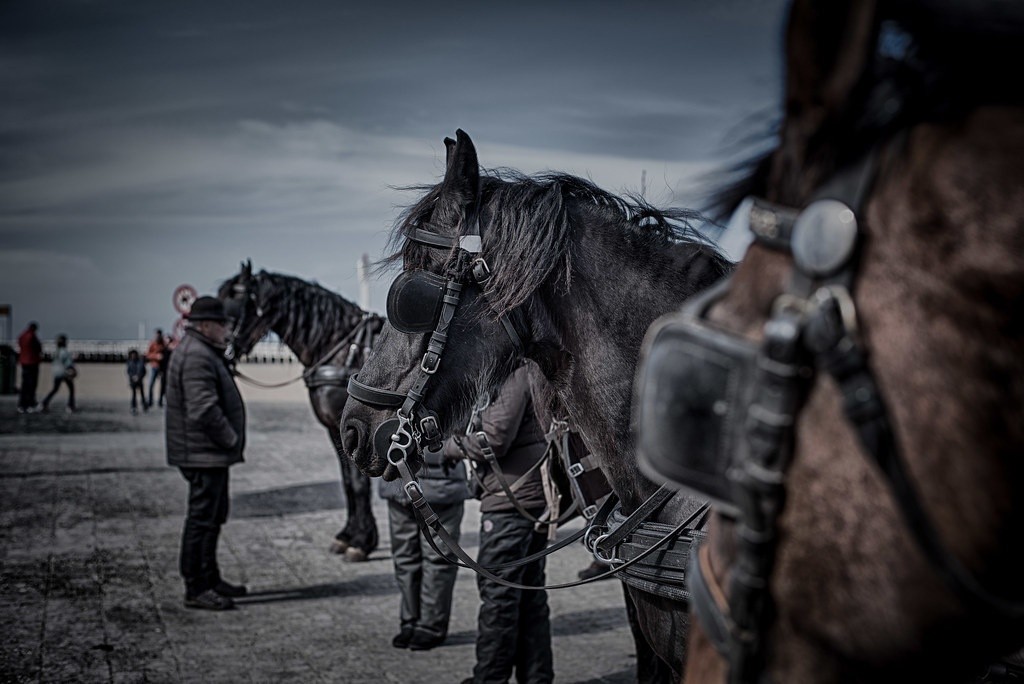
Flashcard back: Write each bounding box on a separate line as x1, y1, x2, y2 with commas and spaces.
217, 257, 386, 563
680, 0, 1024, 684
341, 128, 737, 684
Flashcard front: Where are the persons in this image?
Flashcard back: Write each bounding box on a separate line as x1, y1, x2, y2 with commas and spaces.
38, 335, 78, 413
421, 356, 555, 684
146, 329, 173, 407
16, 321, 43, 413
126, 350, 148, 413
166, 296, 246, 607
0, 346, 20, 395
378, 460, 468, 651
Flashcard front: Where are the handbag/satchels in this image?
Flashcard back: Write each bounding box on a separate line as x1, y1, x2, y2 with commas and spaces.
63, 366, 78, 381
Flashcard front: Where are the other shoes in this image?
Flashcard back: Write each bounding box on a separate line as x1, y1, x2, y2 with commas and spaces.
209, 576, 248, 598
37, 405, 49, 415
183, 589, 234, 610
391, 629, 416, 649
408, 631, 446, 651
66, 406, 82, 415
459, 677, 475, 684
17, 404, 36, 414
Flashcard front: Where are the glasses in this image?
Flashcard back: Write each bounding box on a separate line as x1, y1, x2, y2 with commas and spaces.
208, 316, 228, 327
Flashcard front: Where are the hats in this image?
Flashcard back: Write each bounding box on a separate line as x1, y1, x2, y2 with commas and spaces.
187, 295, 236, 322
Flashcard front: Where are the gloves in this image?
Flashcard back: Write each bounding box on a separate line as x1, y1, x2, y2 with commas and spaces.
438, 452, 460, 477
